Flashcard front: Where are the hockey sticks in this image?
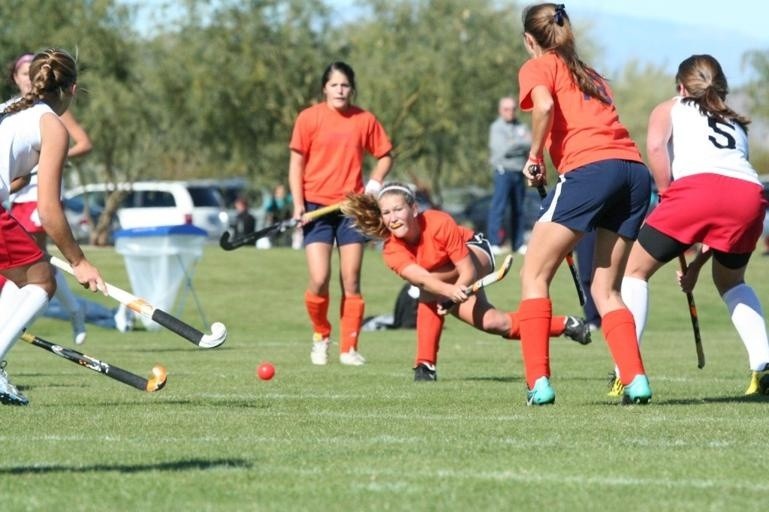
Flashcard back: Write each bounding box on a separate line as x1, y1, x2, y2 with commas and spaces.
679, 253, 705, 368
442, 254, 513, 308
44, 252, 227, 349
220, 201, 351, 250
21, 332, 167, 392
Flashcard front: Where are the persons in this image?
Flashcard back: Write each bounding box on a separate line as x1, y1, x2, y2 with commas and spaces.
229, 199, 256, 245
0, 53, 94, 345
607, 55, 769, 396
340, 182, 591, 382
518, 3, 651, 405
263, 185, 294, 251
487, 97, 532, 256
289, 61, 394, 365
0, 46, 108, 406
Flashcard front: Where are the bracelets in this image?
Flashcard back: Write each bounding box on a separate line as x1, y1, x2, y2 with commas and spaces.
363, 179, 383, 195
529, 156, 543, 163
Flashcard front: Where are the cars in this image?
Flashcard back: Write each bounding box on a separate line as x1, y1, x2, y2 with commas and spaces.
450, 191, 551, 235
223, 174, 280, 234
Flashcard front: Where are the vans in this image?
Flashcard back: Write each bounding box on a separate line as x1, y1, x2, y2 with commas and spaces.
61, 181, 228, 238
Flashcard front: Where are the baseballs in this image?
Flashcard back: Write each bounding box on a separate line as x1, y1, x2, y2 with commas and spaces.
259, 363, 274, 380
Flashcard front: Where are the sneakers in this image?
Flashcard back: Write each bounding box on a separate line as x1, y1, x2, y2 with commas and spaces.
526, 376, 555, 406
311, 333, 330, 366
606, 374, 652, 405
412, 361, 437, 382
72, 308, 88, 345
1, 361, 29, 404
745, 363, 769, 394
114, 303, 133, 332
565, 316, 592, 344
339, 351, 367, 365
